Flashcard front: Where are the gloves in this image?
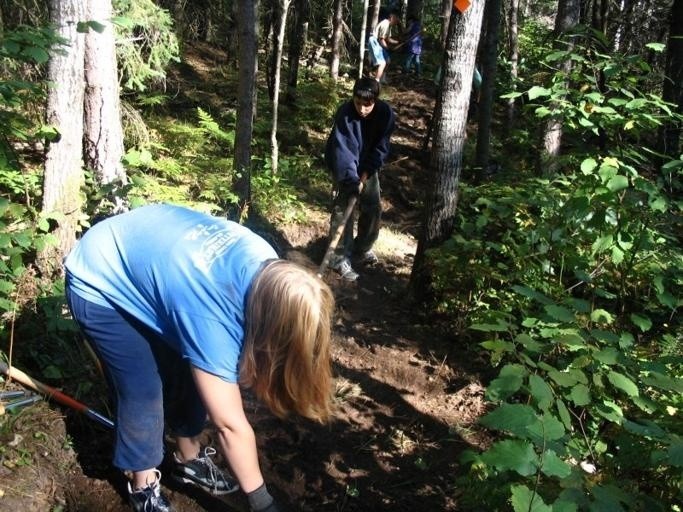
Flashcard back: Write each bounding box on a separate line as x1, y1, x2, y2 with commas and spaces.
246, 485, 283, 511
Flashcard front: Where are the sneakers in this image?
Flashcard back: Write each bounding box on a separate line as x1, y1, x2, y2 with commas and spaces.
352, 246, 380, 266
325, 254, 360, 282
170, 443, 240, 495
127, 478, 175, 512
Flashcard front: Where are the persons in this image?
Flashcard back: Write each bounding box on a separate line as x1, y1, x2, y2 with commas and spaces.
434, 64, 483, 139
325, 78, 394, 281
394, 13, 422, 80
369, 13, 399, 83
64, 203, 335, 512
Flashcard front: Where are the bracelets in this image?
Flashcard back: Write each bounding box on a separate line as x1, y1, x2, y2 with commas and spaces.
246, 482, 275, 512
